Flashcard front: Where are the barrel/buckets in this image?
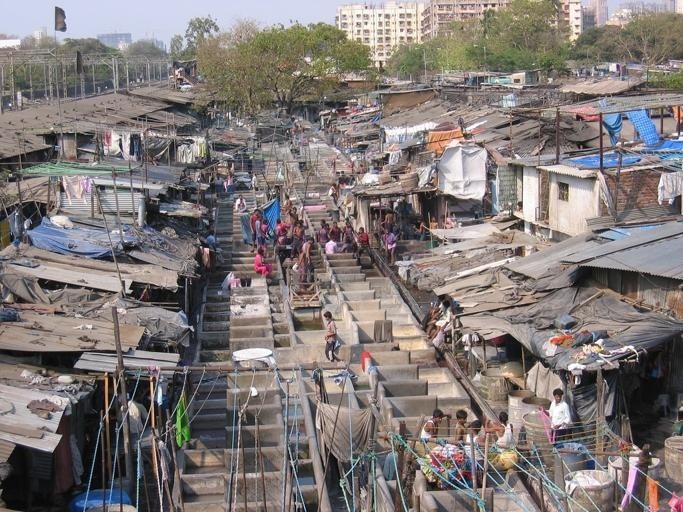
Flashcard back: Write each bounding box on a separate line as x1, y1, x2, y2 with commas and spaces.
554, 444, 586, 489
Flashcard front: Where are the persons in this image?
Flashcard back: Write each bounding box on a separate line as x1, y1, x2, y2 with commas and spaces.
115, 391, 149, 436
453, 409, 468, 441
461, 331, 481, 376
538, 386, 571, 442
328, 182, 338, 200
485, 412, 514, 443
465, 420, 482, 443
236, 195, 247, 213
419, 409, 443, 438
226, 172, 236, 192
206, 229, 218, 273
250, 172, 259, 190
210, 173, 224, 192
249, 194, 460, 363
